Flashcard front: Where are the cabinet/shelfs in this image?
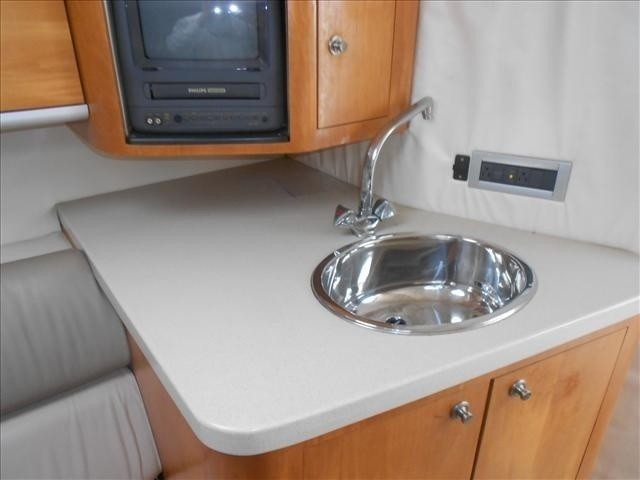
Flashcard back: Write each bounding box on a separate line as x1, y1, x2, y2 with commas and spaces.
122, 315, 638, 480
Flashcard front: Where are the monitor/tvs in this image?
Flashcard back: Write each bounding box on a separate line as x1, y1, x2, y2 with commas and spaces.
111, 0, 287, 135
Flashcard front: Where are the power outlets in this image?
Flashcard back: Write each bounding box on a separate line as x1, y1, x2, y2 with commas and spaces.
467, 148, 573, 205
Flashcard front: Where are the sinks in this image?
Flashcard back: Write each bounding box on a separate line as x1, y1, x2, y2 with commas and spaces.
312, 231, 536, 336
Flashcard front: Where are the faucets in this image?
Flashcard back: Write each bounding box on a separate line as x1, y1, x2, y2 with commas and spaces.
359, 96, 433, 214
